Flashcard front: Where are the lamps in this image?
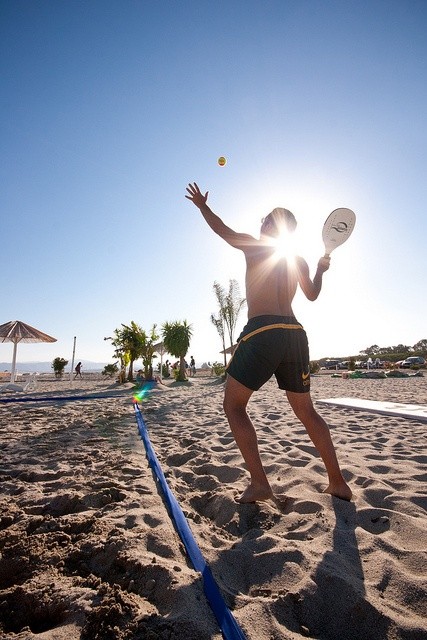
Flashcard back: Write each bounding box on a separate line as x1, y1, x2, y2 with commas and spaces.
1, 372, 36, 394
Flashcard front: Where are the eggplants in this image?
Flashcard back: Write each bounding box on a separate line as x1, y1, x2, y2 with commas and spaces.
321, 207, 356, 257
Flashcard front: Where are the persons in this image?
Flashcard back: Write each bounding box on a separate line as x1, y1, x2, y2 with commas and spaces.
189, 356, 195, 374
183, 359, 187, 375
74, 362, 82, 380
185, 180, 354, 500
166, 360, 171, 372
173, 361, 179, 371
